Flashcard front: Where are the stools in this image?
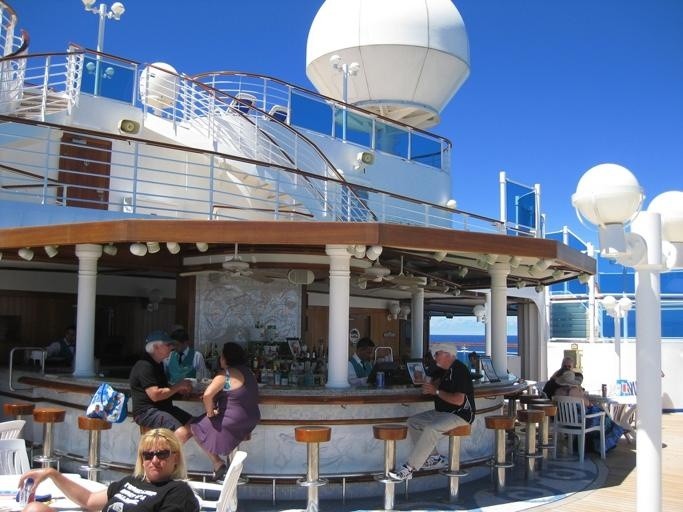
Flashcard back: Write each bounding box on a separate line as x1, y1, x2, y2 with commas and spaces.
439, 423, 473, 504
369, 423, 407, 511
293, 424, 333, 510
1, 399, 251, 486
482, 391, 556, 493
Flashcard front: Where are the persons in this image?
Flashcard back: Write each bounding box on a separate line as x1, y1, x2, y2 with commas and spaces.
467, 352, 480, 370
128, 329, 197, 447
541, 357, 629, 454
16, 427, 201, 511
161, 329, 207, 391
387, 341, 475, 480
191, 342, 258, 482
29, 328, 77, 369
347, 337, 383, 388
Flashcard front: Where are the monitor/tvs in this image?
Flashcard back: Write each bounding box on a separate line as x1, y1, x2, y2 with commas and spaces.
367, 361, 396, 382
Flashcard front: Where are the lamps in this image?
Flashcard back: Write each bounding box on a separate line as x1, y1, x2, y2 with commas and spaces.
386, 303, 411, 321
349, 244, 587, 296
0, 240, 209, 268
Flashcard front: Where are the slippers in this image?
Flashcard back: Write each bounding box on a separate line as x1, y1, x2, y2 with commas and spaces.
210, 464, 226, 481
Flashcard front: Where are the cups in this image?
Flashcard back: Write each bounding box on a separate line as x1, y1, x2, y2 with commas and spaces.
375, 371, 385, 387
182, 376, 198, 393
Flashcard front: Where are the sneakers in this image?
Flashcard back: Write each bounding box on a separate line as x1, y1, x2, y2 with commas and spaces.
421, 454, 447, 470
388, 465, 413, 480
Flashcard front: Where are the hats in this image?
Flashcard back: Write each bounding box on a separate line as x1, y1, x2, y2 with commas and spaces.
430, 343, 459, 359
555, 371, 580, 386
145, 329, 176, 342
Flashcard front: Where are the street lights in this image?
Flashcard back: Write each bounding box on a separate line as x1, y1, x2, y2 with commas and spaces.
569, 163, 683, 512
85, 62, 115, 96
81, 0, 126, 98
329, 54, 361, 143
601, 294, 633, 402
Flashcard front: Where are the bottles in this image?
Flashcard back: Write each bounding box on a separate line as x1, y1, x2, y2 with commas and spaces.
14, 478, 36, 505
203, 342, 220, 371
602, 384, 607, 398
244, 323, 327, 387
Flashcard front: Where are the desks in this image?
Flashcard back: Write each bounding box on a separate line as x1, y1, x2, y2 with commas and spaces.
582, 394, 639, 445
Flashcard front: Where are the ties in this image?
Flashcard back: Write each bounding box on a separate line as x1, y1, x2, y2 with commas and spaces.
178, 352, 184, 364
360, 360, 366, 369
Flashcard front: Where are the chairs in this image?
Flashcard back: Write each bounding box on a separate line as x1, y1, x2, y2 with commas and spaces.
526, 379, 605, 464
212, 90, 257, 116
0, 420, 247, 511
260, 103, 291, 124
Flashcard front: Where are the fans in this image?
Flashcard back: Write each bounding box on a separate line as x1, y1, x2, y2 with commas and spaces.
175, 241, 289, 284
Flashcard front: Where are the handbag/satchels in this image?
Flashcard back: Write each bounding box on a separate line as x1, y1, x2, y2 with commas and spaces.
86, 384, 127, 423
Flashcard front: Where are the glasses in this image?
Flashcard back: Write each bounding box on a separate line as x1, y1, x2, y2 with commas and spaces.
143, 450, 176, 460
